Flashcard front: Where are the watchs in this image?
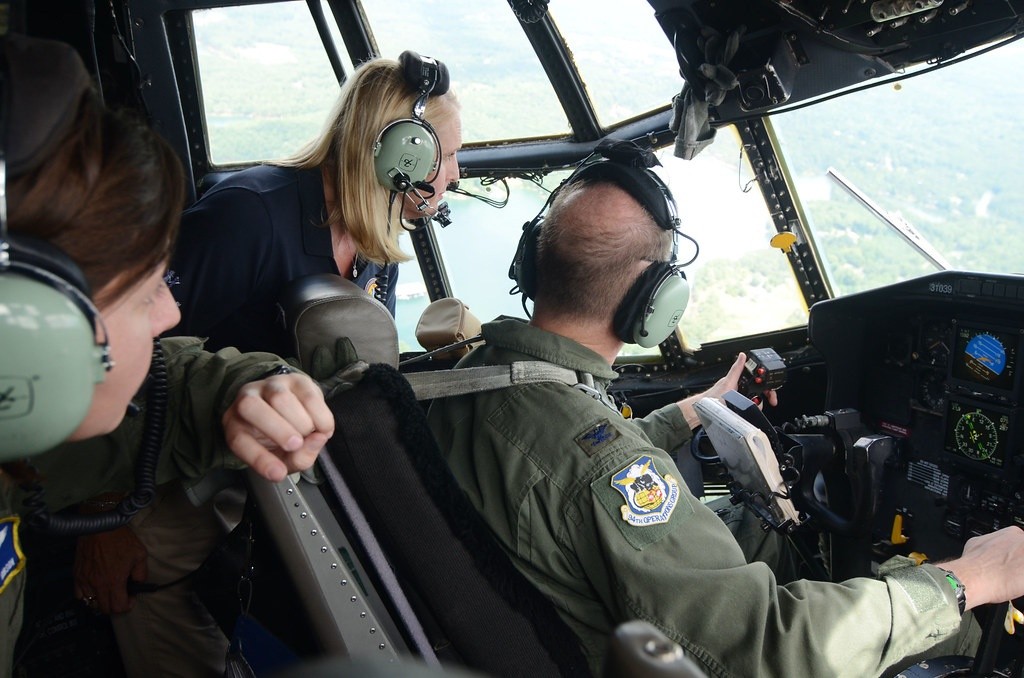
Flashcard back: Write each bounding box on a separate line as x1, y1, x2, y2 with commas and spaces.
935, 566, 967, 616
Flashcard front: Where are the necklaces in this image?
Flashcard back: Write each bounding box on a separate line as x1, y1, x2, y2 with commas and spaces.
344, 223, 360, 277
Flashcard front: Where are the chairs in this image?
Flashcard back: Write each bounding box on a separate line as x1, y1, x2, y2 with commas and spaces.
248, 271, 596, 678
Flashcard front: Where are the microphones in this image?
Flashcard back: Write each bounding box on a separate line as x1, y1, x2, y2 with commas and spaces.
387, 167, 452, 227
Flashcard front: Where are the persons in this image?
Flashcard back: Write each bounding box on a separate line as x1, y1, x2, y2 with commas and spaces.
67, 54, 460, 678
0, 34, 335, 678
423, 159, 1024, 678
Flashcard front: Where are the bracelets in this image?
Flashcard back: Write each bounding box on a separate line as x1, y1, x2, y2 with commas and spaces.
254, 364, 291, 381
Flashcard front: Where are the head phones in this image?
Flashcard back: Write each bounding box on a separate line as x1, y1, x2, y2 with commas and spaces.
509, 138, 700, 347
0, 37, 114, 463
374, 50, 450, 193
